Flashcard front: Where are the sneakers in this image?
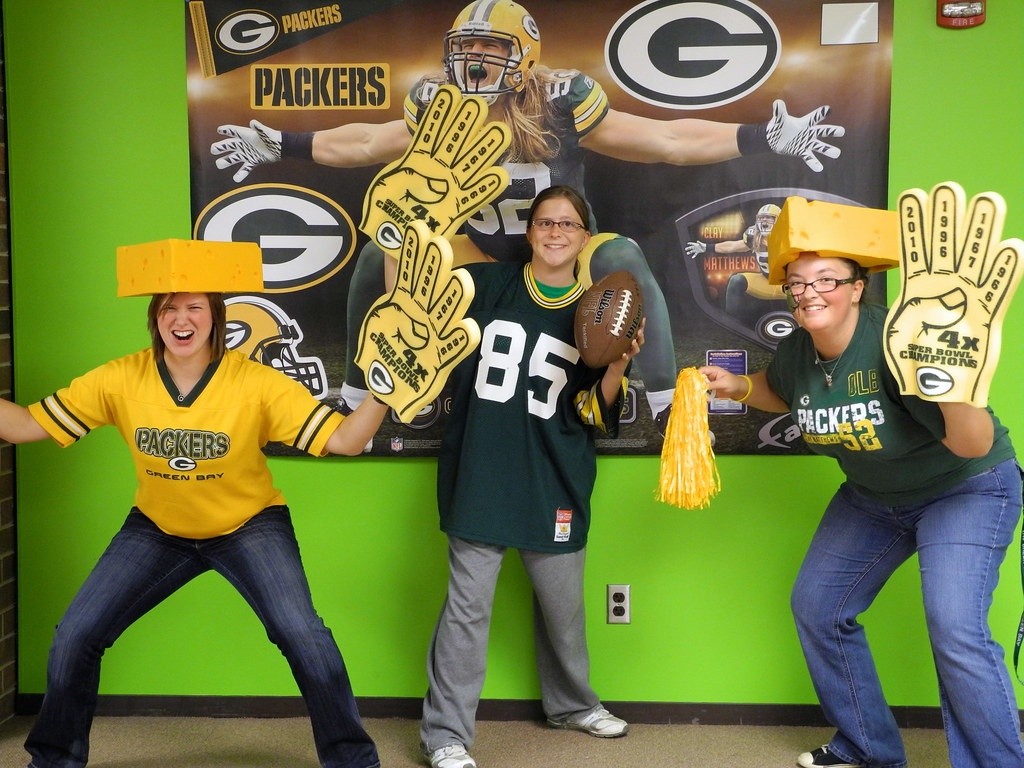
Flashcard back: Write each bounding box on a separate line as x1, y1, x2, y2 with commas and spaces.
797, 744, 866, 768
547, 708, 628, 737
422, 744, 476, 768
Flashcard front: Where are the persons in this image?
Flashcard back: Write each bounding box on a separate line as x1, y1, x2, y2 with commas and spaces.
0, 292, 390, 768
698, 251, 1024, 768
211, 0, 845, 452
384, 185, 645, 768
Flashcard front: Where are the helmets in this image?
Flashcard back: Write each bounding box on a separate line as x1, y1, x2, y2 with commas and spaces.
449, 0, 541, 92
757, 204, 781, 223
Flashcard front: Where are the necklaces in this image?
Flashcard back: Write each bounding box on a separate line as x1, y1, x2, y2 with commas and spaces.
166, 367, 194, 402
816, 351, 844, 386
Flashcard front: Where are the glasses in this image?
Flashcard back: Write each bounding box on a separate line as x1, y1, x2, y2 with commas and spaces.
782, 277, 858, 297
531, 220, 585, 233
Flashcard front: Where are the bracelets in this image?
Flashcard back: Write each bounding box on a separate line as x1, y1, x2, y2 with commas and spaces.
731, 374, 753, 402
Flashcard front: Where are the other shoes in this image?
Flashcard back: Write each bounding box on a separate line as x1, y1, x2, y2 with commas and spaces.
325, 397, 374, 453
654, 405, 715, 447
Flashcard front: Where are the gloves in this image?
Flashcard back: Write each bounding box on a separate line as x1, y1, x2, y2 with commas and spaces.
211, 120, 312, 183
685, 240, 715, 259
737, 99, 846, 172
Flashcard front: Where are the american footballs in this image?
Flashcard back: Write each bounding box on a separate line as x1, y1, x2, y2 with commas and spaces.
574, 271, 644, 369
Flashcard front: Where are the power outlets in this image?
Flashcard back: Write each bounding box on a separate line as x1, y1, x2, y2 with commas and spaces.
607, 584, 630, 625
